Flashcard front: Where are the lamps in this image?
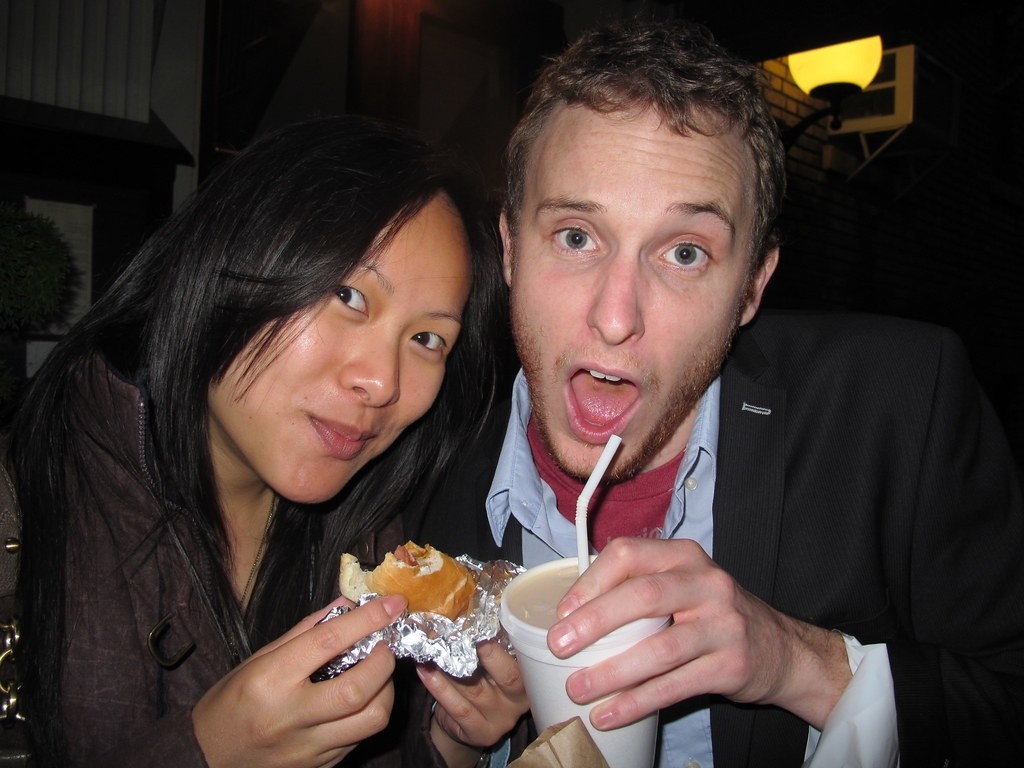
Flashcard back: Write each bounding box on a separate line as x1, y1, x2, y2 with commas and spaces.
774, 35, 885, 154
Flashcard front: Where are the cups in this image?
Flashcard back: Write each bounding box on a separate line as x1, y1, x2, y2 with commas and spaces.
497, 555, 675, 768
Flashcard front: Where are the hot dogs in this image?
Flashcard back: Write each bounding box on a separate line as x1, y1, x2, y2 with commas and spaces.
339, 539, 476, 622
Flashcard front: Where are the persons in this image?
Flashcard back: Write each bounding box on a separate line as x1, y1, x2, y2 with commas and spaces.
1, 116, 532, 768
399, 8, 1024, 768
